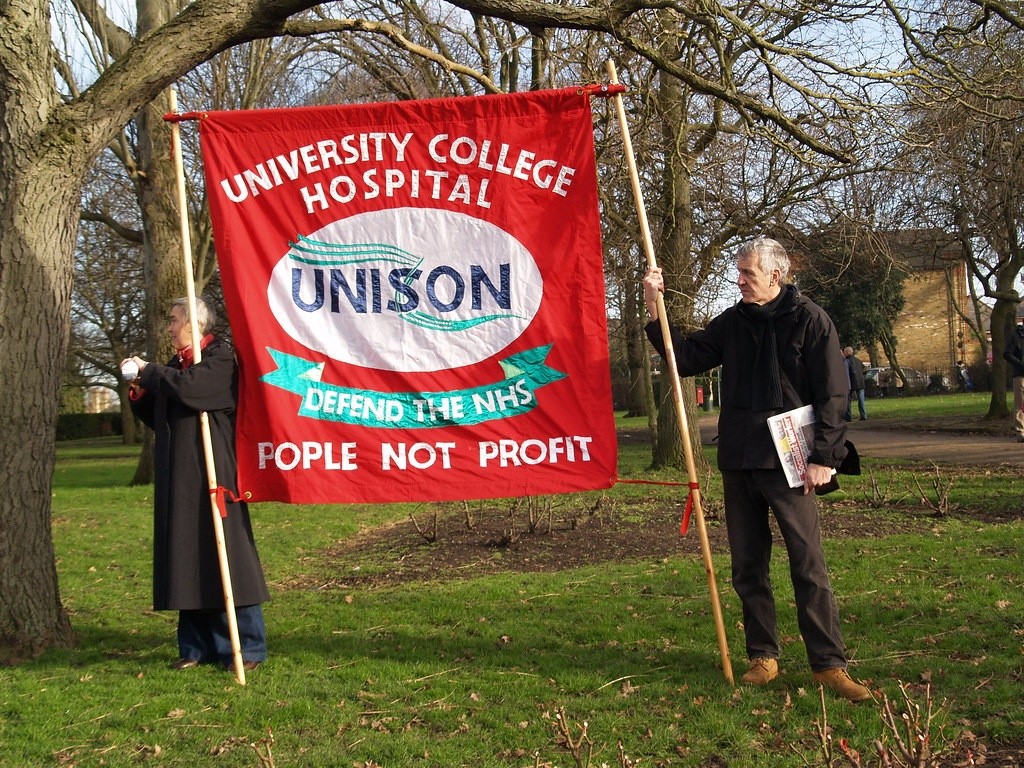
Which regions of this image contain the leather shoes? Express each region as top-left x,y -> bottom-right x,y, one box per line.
226,660 -> 260,673
168,657 -> 200,671
741,657 -> 779,687
813,667 -> 870,702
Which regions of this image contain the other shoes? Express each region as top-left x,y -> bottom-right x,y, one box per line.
859,418 -> 867,421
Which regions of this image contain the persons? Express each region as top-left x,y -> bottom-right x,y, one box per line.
642,238 -> 876,704
877,367 -> 906,399
840,347 -> 868,422
1002,318 -> 1024,443
119,294 -> 275,672
957,360 -> 964,383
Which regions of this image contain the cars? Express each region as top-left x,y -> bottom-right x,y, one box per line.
864,367 -> 950,400
